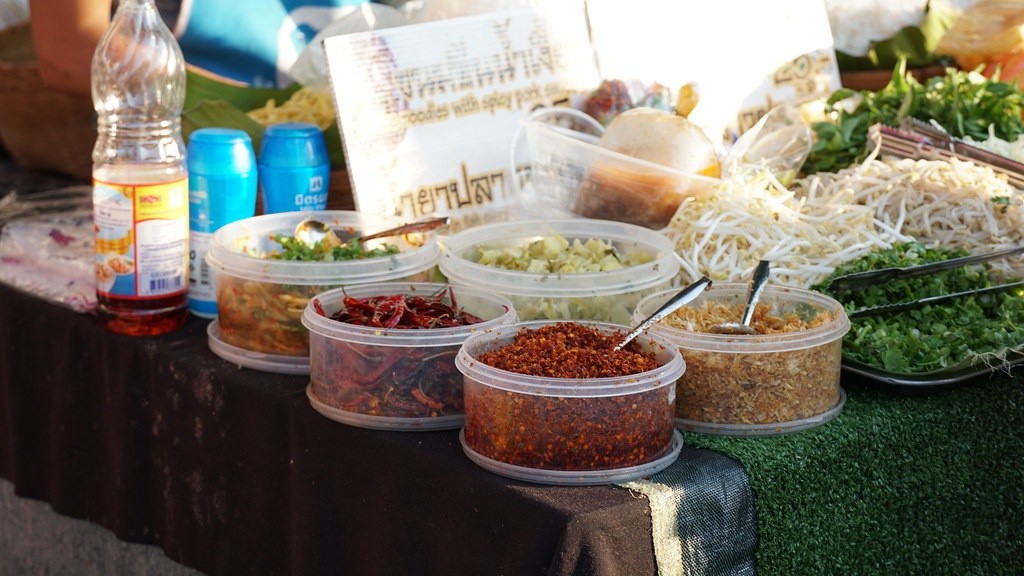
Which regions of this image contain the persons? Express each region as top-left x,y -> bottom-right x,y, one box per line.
30,0 -> 496,96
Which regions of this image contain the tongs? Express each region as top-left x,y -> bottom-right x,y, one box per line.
825,245 -> 1024,318
866,116 -> 1024,191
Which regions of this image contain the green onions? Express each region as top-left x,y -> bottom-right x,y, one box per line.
269,235 -> 400,261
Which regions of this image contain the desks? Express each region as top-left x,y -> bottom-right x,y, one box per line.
0,154 -> 1024,576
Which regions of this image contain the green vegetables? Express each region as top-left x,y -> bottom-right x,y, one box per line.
808,241 -> 1024,372
801,55 -> 1024,172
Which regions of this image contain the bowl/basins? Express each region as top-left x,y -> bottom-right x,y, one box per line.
438,218 -> 682,325
633,281 -> 851,437
300,281 -> 521,433
525,119 -> 724,230
454,319 -> 684,486
205,209 -> 442,375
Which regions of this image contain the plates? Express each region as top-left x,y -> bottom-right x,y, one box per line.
841,352 -> 1024,387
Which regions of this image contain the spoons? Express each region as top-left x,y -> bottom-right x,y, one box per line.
709,259 -> 771,336
294,217 -> 448,252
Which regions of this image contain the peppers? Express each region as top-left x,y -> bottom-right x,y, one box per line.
304,284 -> 486,416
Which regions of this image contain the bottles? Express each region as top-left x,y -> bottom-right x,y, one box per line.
259,122 -> 331,214
184,126 -> 259,319
89,0 -> 189,337
